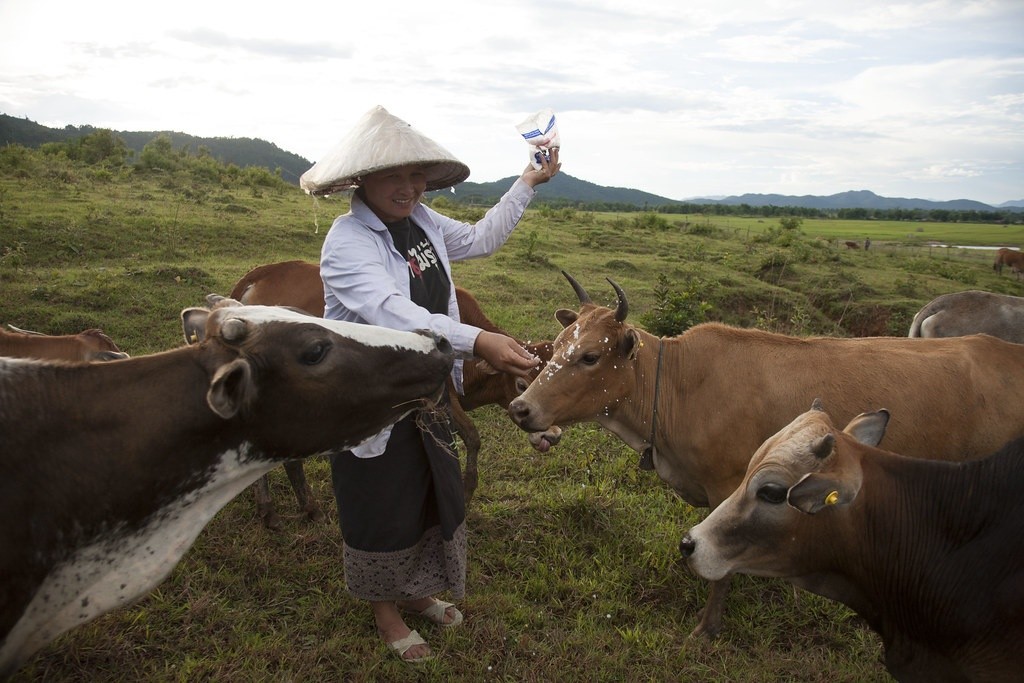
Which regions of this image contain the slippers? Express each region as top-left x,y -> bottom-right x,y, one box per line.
376,625 -> 436,663
397,598 -> 464,628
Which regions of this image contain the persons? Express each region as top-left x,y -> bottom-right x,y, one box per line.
300,106 -> 561,663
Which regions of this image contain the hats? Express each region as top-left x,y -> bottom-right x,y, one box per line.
300,105 -> 470,196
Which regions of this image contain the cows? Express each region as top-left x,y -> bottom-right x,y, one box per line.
0,242 -> 1023,683
